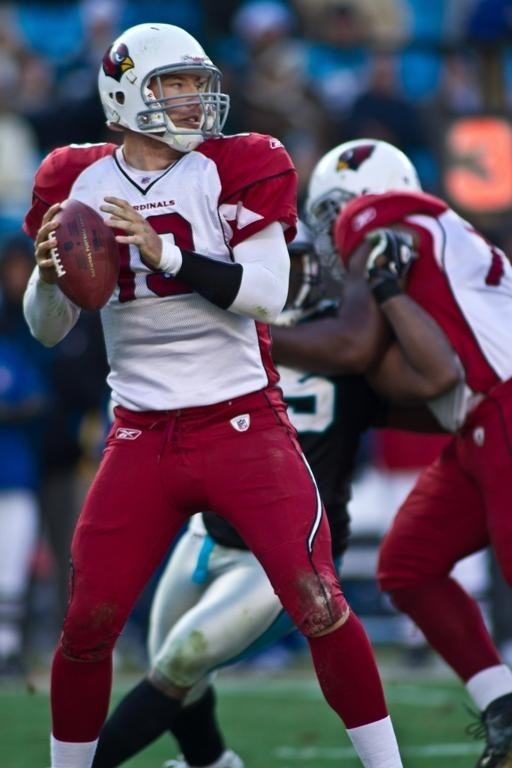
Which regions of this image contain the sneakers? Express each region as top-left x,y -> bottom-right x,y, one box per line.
462,691 -> 511,767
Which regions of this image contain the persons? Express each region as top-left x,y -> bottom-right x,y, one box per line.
92,211 -> 463,768
22,22 -> 402,768
269,139 -> 512,768
1,1 -> 512,677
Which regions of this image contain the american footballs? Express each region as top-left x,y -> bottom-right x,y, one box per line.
46,198 -> 120,312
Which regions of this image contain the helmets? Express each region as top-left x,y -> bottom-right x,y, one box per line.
281,218 -> 320,315
97,22 -> 230,153
302,137 -> 425,279
